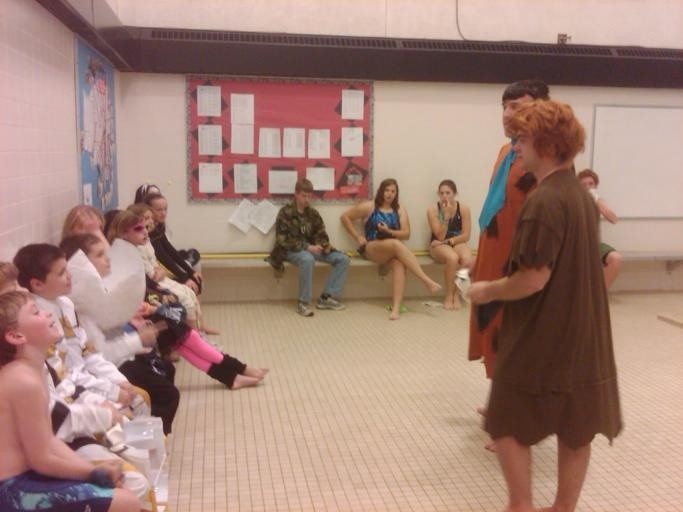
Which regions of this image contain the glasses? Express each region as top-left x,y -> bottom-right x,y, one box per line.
129,223 -> 150,231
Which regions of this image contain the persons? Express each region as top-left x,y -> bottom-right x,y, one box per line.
0,184 -> 270,512
340,178 -> 443,319
262,178 -> 352,317
466,79 -> 551,454
467,99 -> 622,511
576,169 -> 624,291
426,179 -> 473,309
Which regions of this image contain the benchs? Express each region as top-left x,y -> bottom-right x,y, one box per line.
189,247 -> 683,277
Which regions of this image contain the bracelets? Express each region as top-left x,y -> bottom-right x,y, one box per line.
448,237 -> 455,246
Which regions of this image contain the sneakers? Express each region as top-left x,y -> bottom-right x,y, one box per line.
296,301 -> 314,317
317,295 -> 346,311
